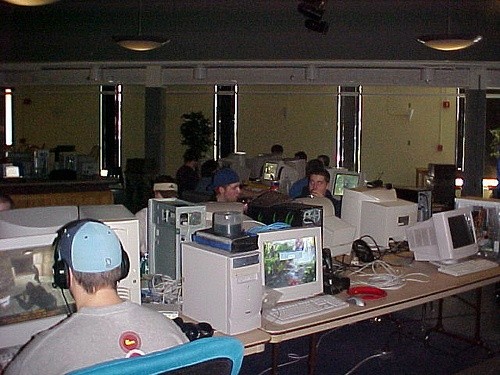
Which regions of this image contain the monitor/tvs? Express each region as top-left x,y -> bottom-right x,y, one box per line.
296,196 -> 357,257
406,207 -> 478,265
249,154 -> 277,180
331,172 -> 366,196
262,161 -> 286,181
218,153 -> 247,182
0,232 -> 77,326
255,226 -> 323,302
339,186 -> 418,251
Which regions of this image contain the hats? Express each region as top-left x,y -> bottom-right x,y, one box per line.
213,168 -> 240,186
56,221 -> 123,273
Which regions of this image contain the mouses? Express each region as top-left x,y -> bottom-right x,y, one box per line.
345,296 -> 366,307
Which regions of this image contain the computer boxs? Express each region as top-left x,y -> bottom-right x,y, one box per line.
289,158 -> 306,180
395,186 -> 432,220
454,196 -> 500,251
78,204 -> 142,305
148,197 -> 206,302
179,243 -> 262,335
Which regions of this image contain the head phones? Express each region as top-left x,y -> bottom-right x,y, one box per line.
322,273 -> 351,295
173,318 -> 213,341
351,235 -> 381,263
53,217 -> 129,289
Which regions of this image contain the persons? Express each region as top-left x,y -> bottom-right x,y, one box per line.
299,168 -> 341,218
197,159 -> 221,195
212,169 -> 249,212
318,155 -> 330,166
271,145 -> 283,156
294,151 -> 307,161
1,219 -> 191,375
176,149 -> 201,191
134,175 -> 183,257
290,160 -> 331,197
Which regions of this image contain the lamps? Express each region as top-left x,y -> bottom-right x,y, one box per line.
90,68 -> 103,81
420,68 -> 435,82
193,67 -> 207,80
297,0 -> 329,35
417,0 -> 483,51
306,67 -> 320,82
112,0 -> 171,51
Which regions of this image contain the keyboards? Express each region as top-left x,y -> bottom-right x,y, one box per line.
262,295 -> 349,325
438,258 -> 499,276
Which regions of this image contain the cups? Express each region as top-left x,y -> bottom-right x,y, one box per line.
483,190 -> 492,200
456,190 -> 461,198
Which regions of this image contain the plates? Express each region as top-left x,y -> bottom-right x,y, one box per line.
347,286 -> 387,300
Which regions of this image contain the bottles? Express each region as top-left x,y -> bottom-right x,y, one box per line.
140,253 -> 149,297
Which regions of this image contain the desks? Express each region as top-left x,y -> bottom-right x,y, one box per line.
259,251 -> 500,375
